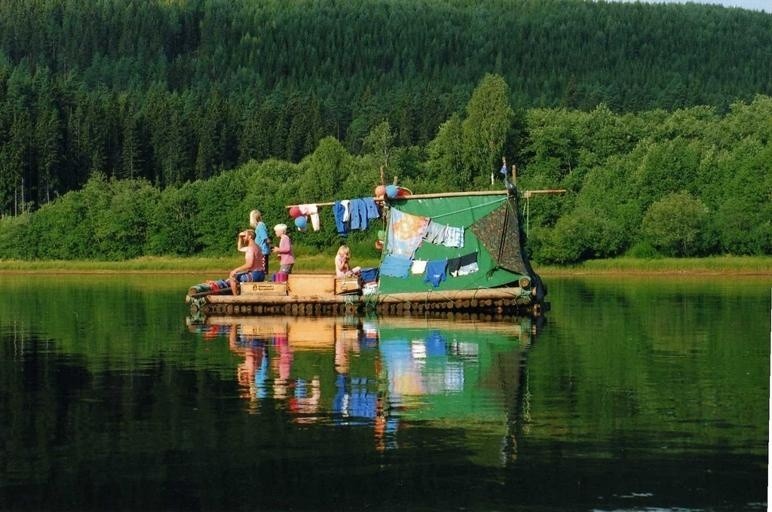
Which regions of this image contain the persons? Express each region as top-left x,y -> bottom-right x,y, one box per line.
229,209 -> 295,296
335,245 -> 361,279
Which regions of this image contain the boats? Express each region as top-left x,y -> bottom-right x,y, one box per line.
185,157 -> 567,317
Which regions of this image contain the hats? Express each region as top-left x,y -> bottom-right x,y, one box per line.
374,185 -> 397,198
289,207 -> 306,232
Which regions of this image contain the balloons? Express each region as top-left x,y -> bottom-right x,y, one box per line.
289,207 -> 306,228
375,185 -> 397,198
